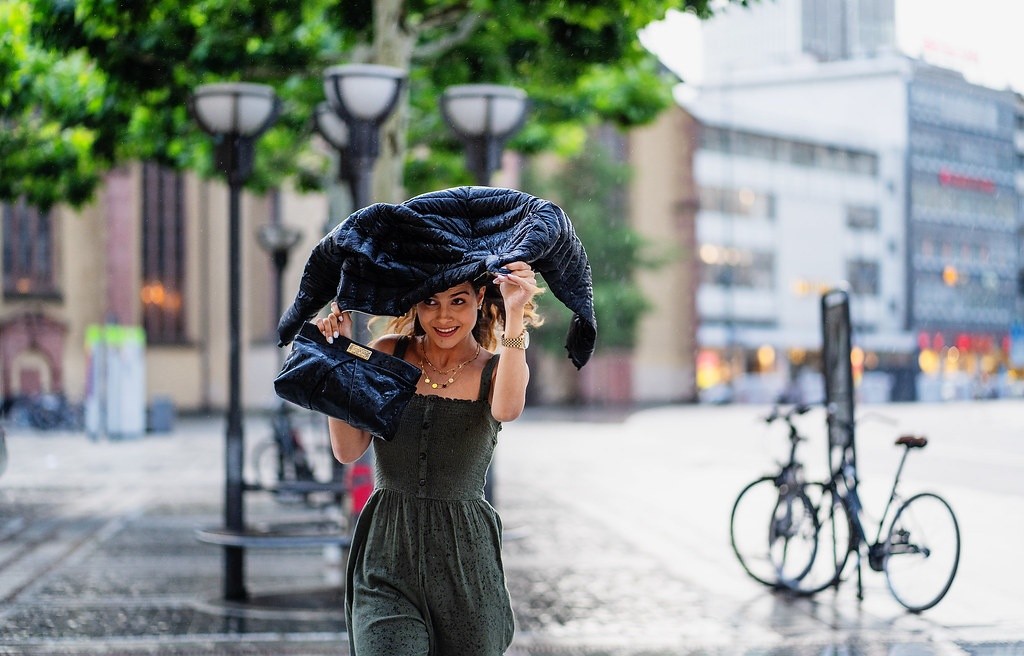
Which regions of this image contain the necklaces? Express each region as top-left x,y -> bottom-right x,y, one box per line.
418,334 -> 480,390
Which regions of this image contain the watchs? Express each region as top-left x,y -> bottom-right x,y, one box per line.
501,328 -> 530,351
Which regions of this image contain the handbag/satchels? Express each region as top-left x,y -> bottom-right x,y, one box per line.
274,322 -> 422,441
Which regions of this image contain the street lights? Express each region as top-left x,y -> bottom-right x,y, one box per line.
438,83 -> 529,190
188,79 -> 280,605
311,63 -> 411,508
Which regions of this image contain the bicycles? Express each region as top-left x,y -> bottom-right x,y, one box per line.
729,405 -> 963,615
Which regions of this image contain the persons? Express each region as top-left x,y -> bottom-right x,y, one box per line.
315,260 -> 539,656
